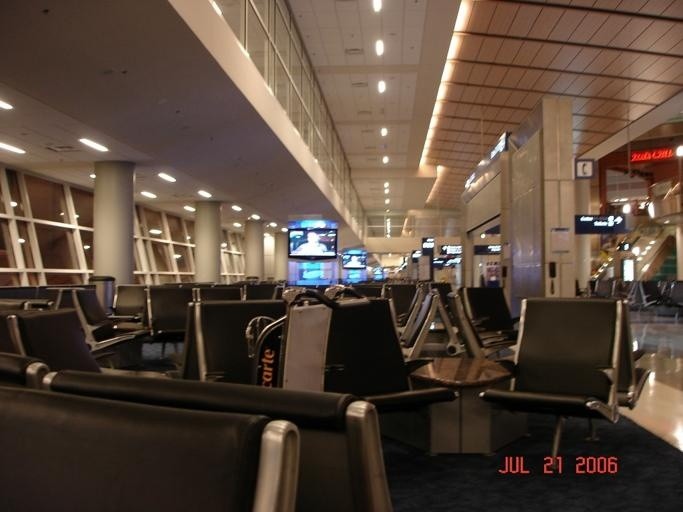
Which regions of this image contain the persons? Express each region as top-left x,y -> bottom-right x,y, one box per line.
343,255 -> 364,268
293,230 -> 328,255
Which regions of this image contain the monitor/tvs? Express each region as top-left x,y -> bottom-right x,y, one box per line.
287,229 -> 338,260
342,253 -> 367,269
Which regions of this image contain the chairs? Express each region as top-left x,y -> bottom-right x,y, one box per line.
1,277 -> 683,512
